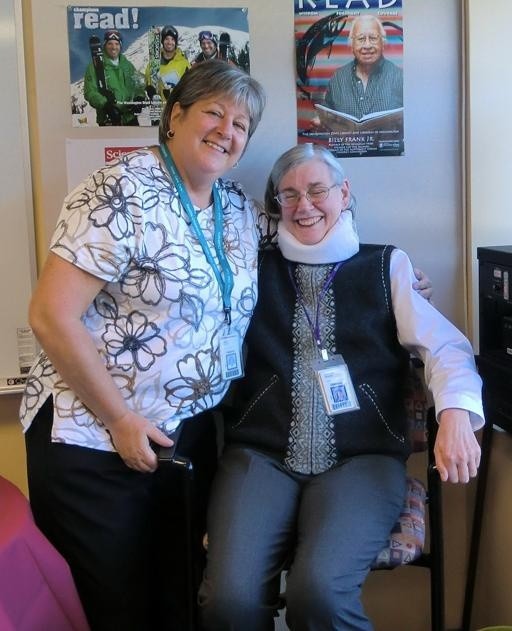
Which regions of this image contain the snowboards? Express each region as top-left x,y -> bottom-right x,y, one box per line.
219,33 -> 230,63
149,25 -> 163,125
90,35 -> 114,125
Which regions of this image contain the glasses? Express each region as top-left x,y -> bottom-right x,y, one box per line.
274,183 -> 337,208
105,31 -> 120,40
198,31 -> 213,40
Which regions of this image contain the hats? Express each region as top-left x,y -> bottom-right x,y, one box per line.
161,25 -> 178,44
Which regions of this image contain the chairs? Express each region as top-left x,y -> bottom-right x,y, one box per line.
172,357 -> 446,630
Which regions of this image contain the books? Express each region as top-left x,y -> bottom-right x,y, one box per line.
314,103 -> 403,140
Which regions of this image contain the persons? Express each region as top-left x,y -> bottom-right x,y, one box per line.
309,16 -> 403,133
84,30 -> 145,126
190,31 -> 235,65
145,24 -> 192,111
194,141 -> 487,631
20,60 -> 434,631
